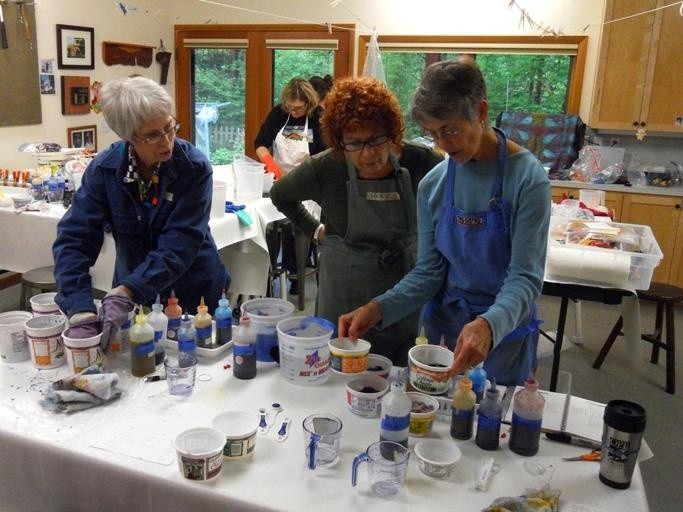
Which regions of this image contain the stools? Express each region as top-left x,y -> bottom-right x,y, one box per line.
20,265 -> 56,309
592,281 -> 683,394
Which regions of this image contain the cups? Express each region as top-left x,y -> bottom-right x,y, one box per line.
303,412 -> 343,473
351,440 -> 411,497
163,351 -> 197,396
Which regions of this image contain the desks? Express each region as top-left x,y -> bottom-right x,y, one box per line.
0,156 -> 309,294
539,202 -> 639,393
0,298 -> 647,512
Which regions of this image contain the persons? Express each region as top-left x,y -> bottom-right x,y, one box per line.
309,74 -> 333,109
254,76 -> 329,296
270,78 -> 446,367
338,60 -> 550,386
52,76 -> 230,355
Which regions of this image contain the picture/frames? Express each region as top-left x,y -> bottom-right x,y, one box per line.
67,125 -> 98,153
56,23 -> 95,70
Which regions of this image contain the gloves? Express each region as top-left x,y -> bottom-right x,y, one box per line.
260,154 -> 281,179
68,316 -> 99,339
99,295 -> 134,353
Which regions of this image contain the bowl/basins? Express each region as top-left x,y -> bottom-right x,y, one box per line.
405,392 -> 439,436
328,337 -> 370,376
369,353 -> 394,376
414,439 -> 461,481
12,196 -> 32,211
174,427 -> 227,483
212,411 -> 257,460
344,375 -> 389,420
407,344 -> 456,395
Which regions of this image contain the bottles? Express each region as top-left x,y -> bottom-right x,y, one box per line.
48,175 -> 57,202
57,172 -> 65,202
42,174 -> 48,200
475,377 -> 502,451
32,176 -> 42,199
509,368 -> 545,456
193,296 -> 213,350
450,378 -> 476,440
64,179 -> 71,209
215,288 -> 232,344
147,293 -> 168,365
177,309 -> 196,368
469,361 -> 487,415
232,308 -> 257,379
129,304 -> 155,377
379,370 -> 412,462
165,289 -> 182,341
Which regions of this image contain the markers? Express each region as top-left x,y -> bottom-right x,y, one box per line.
546,431 -> 602,449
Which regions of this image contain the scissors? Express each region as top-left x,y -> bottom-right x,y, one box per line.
561,448 -> 604,461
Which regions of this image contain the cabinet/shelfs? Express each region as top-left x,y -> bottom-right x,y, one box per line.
548,186 -> 683,287
578,0 -> 683,134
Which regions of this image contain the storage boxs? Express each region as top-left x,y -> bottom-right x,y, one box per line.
546,217 -> 664,290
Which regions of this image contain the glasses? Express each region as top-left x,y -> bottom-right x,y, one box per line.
339,133 -> 389,152
420,128 -> 458,142
285,102 -> 307,112
133,118 -> 181,145
